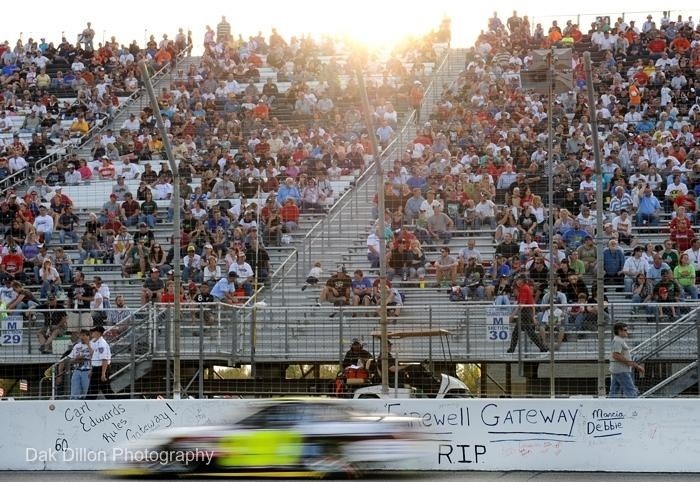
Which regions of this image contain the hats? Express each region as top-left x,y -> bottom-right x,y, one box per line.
90,325 -> 105,333
0,10 -> 700,302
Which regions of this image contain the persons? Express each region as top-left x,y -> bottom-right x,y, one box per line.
609,323 -> 646,397
341,338 -> 413,388
68,326 -> 115,400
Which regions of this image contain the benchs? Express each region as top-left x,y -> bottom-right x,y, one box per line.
694,220 -> 700,359
